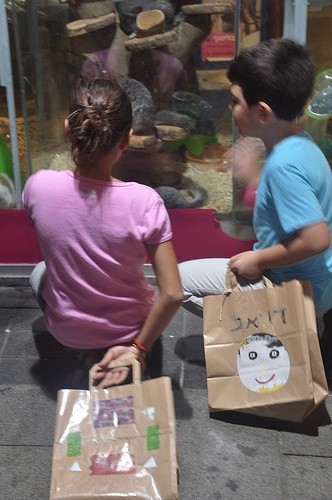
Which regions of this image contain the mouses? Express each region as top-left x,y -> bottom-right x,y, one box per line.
118,78 -> 156,134
154,184 -> 208,209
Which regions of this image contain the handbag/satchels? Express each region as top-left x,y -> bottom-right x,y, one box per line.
48,360 -> 191,497
202,268 -> 329,422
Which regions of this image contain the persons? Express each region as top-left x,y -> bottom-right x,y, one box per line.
177,40 -> 332,341
21,76 -> 182,389
76,13 -> 188,115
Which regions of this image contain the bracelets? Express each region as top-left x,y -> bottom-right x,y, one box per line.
130,338 -> 151,353
